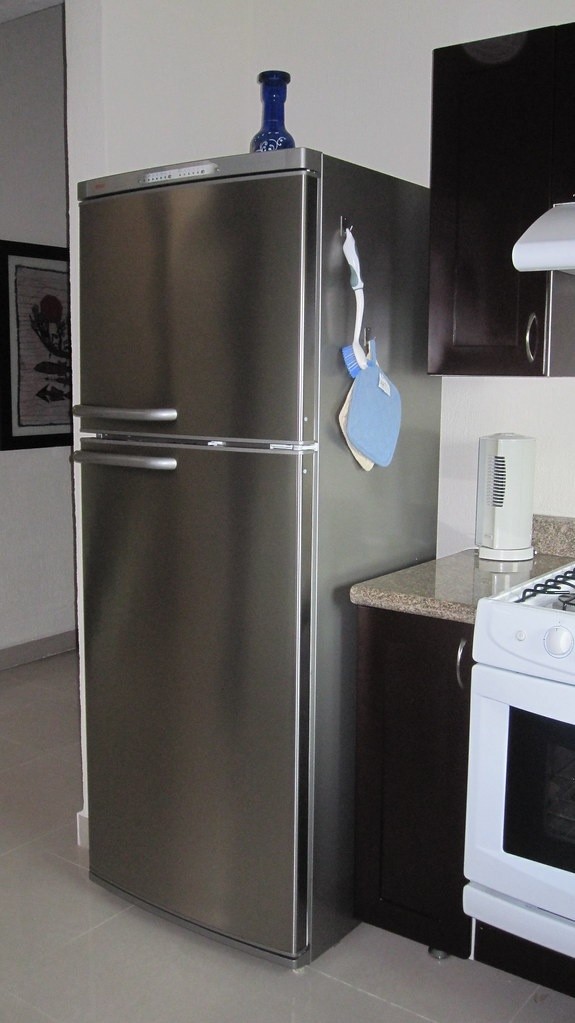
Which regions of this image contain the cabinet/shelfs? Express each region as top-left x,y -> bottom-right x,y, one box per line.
426,24 -> 574,378
355,604 -> 473,962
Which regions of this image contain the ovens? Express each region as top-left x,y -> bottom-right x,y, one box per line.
463,660 -> 575,964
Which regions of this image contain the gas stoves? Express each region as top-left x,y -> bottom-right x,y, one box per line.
470,554 -> 575,687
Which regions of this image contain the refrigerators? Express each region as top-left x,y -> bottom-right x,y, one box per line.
74,145 -> 438,975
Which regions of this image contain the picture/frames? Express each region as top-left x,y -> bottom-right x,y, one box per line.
0,240 -> 73,451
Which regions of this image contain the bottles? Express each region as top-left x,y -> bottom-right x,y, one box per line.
248,68 -> 295,153
